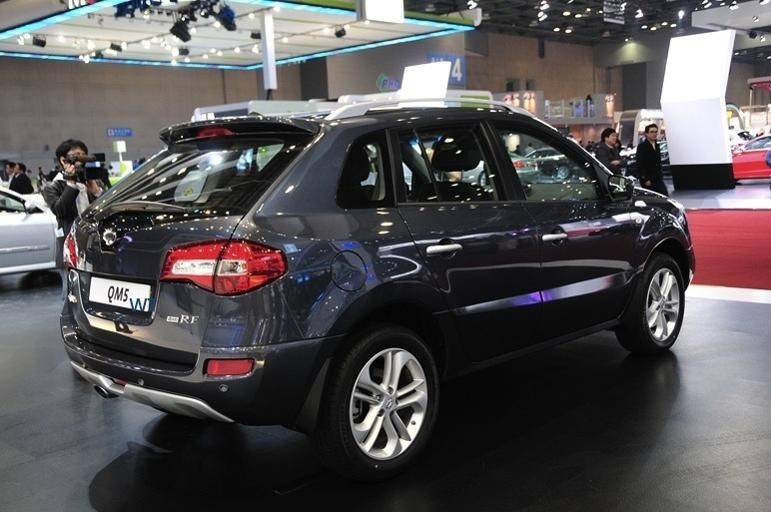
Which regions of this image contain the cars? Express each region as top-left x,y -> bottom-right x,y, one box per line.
527,144 -> 595,182
726,127 -> 771,181
619,136 -> 672,171
449,148 -> 541,188
0,185 -> 72,272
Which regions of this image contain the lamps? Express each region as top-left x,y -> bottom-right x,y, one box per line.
170,0 -> 242,42
24,37 -> 190,59
114,0 -> 173,22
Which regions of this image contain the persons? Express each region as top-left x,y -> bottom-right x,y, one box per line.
0,139 -> 113,301
512,94 -> 741,197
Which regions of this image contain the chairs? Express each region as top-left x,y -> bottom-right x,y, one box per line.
416,129 -> 491,200
335,144 -> 377,209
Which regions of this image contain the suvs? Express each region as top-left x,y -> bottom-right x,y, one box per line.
58,90 -> 701,479
